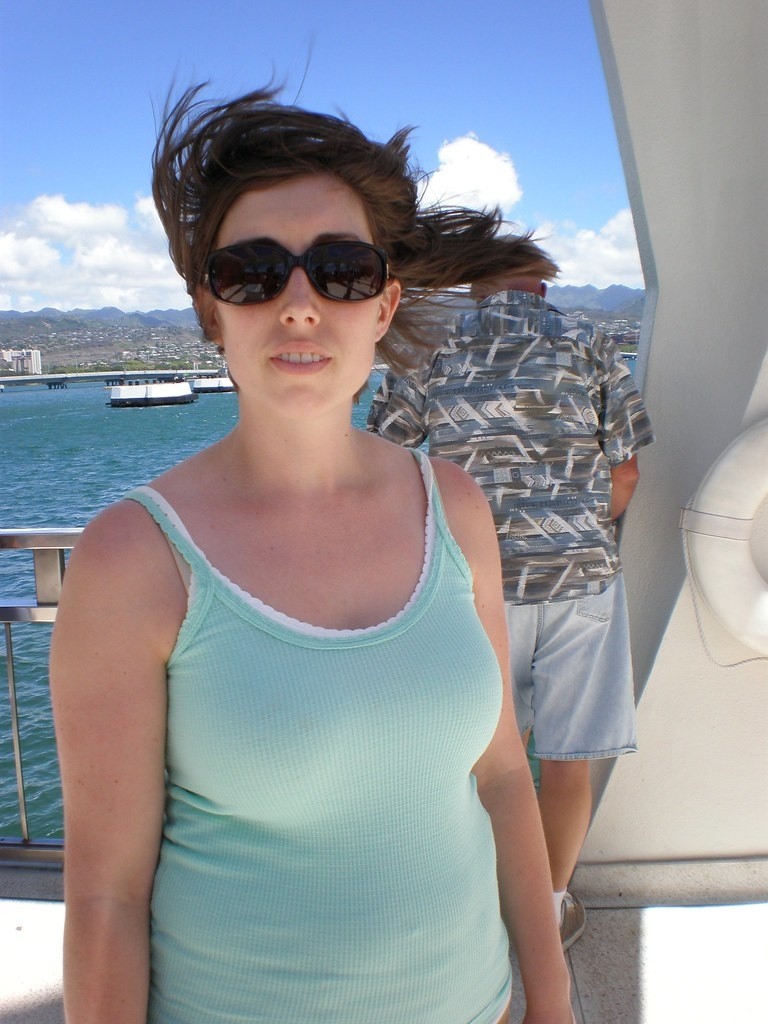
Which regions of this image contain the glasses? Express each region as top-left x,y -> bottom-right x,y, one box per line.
205,240 -> 389,306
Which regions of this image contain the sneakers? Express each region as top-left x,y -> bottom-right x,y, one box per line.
557,887 -> 587,959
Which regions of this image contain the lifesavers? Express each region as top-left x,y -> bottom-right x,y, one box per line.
676,417 -> 768,661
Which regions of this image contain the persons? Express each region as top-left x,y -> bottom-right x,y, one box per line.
362,234 -> 655,955
47,72 -> 571,1023
